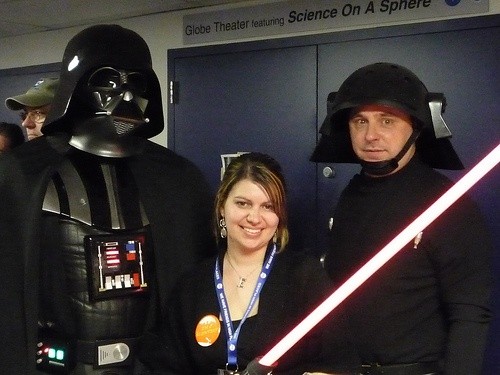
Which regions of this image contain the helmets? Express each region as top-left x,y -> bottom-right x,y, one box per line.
40,23 -> 166,160
308,61 -> 465,170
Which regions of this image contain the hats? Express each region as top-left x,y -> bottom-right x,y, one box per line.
5,76 -> 61,111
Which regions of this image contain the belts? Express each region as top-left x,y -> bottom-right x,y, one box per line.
75,334 -> 154,367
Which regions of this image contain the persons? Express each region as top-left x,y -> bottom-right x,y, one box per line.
155,154 -> 355,375
309,62 -> 491,375
0,25 -> 212,375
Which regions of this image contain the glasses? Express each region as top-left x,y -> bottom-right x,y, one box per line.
22,112 -> 47,123
